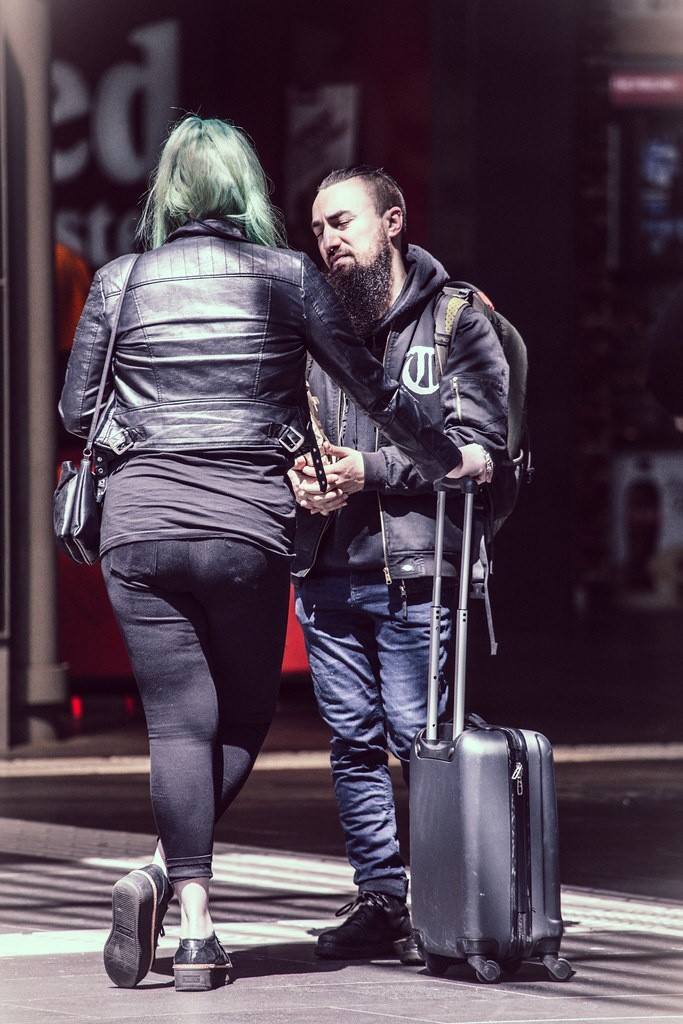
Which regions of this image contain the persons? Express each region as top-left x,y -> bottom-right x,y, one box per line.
285,164 -> 514,964
60,118 -> 495,991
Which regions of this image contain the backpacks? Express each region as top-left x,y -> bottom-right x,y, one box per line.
432,286 -> 526,541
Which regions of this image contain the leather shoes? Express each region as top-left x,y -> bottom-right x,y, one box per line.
172,933 -> 236,990
314,889 -> 412,959
104,864 -> 173,988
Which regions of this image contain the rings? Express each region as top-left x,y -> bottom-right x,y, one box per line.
487,460 -> 494,474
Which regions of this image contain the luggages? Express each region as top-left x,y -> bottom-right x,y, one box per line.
411,476 -> 577,983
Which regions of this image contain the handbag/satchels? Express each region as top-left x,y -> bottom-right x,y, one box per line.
51,459 -> 101,568
